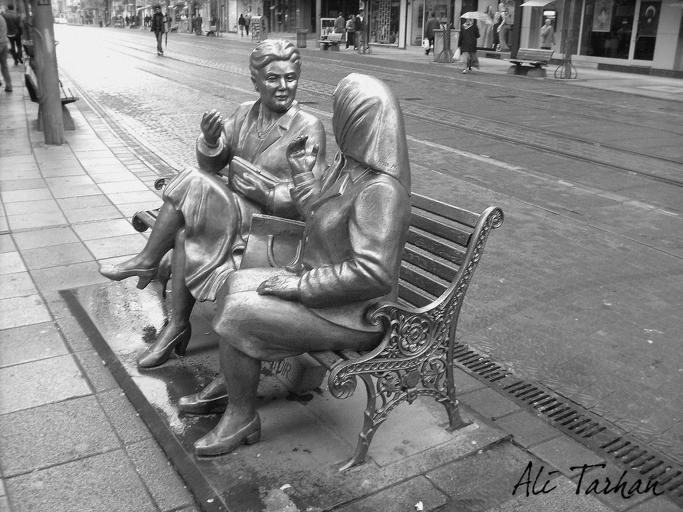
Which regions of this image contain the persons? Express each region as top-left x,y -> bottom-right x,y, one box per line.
238,14 -> 245,25
539,18 -> 556,50
424,11 -> 440,56
333,8 -> 364,49
482,2 -> 511,52
99,38 -> 328,369
150,4 -> 165,55
167,11 -> 202,33
0,15 -> 13,92
125,13 -> 152,27
458,18 -> 480,74
175,75 -> 411,458
244,15 -> 250,35
4,3 -> 23,65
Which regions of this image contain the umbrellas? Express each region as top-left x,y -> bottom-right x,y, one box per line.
460,11 -> 491,22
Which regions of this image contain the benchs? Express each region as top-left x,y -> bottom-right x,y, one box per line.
131,173 -> 506,471
502,47 -> 554,77
317,33 -> 343,51
24,56 -> 79,130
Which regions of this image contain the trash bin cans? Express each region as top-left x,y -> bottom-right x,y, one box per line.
296,26 -> 309,48
